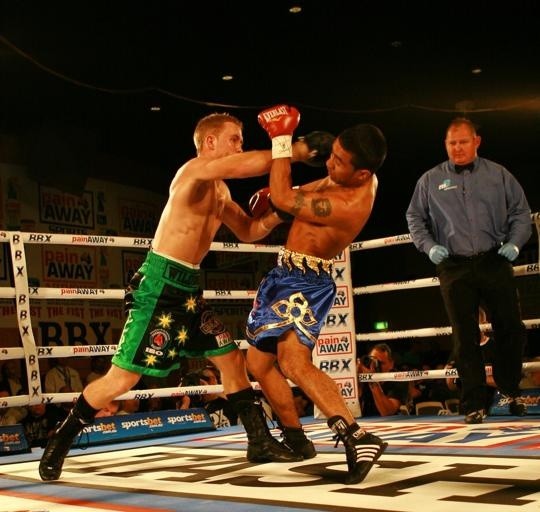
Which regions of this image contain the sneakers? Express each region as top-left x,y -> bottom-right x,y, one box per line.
509,397 -> 527,417
464,408 -> 488,424
276,420 -> 317,459
327,415 -> 388,484
39,403 -> 95,481
234,401 -> 303,462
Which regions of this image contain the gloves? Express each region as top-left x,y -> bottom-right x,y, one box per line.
249,185 -> 300,222
258,105 -> 301,140
498,242 -> 520,261
428,245 -> 448,265
298,131 -> 336,168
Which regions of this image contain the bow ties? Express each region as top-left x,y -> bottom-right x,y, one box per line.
455,163 -> 476,175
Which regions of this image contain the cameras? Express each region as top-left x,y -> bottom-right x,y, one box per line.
360,354 -> 381,372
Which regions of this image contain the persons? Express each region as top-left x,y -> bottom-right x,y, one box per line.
406,120 -> 531,425
39,114 -> 342,481
9,340 -> 540,419
250,106 -> 388,485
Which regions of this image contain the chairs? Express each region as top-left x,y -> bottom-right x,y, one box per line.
416,398 -> 460,417
